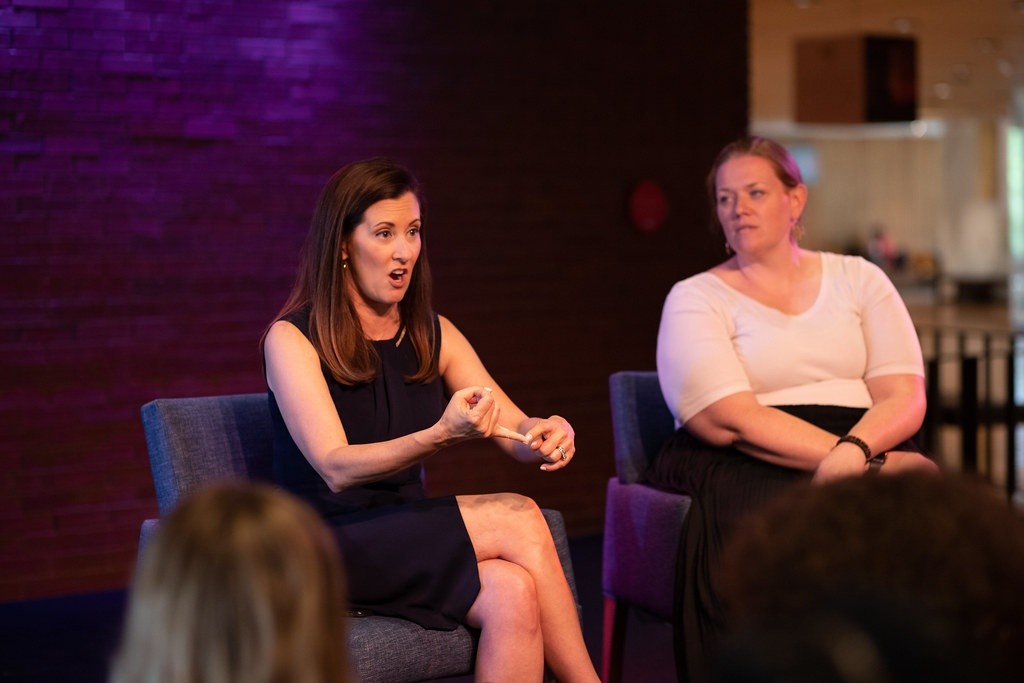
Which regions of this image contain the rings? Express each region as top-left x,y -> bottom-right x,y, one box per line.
556,446 -> 566,460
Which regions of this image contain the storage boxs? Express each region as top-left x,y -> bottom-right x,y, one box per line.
789,33 -> 919,127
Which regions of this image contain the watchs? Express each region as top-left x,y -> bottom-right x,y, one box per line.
868,451 -> 887,470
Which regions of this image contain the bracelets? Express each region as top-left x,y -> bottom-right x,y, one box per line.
836,435 -> 871,459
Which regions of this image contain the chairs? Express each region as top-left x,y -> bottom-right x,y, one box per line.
136,393 -> 576,683
602,371 -> 707,683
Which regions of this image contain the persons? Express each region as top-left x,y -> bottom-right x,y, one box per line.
647,134 -> 937,683
690,467 -> 1024,683
259,158 -> 600,683
111,482 -> 346,683
